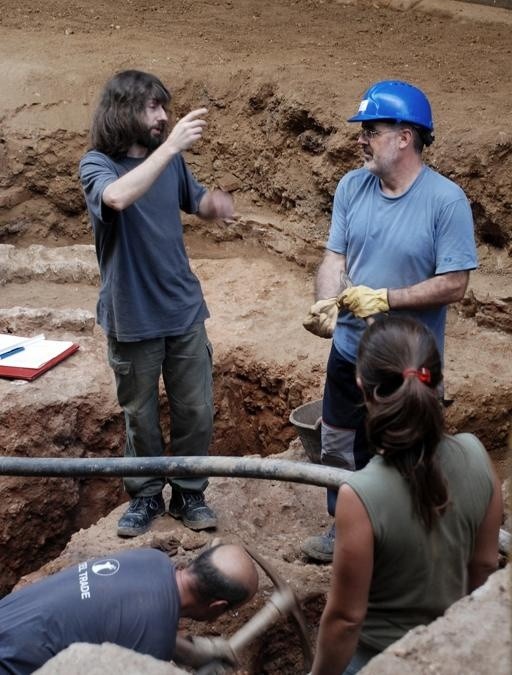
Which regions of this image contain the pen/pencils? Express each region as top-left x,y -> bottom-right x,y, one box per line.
0,345 -> 26,359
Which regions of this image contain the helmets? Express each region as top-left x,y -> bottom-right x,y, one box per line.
348,80 -> 433,132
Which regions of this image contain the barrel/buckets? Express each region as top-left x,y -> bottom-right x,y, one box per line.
290,399 -> 323,464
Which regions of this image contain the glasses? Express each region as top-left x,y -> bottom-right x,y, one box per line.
354,128 -> 392,138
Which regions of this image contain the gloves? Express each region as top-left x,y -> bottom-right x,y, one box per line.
340,284 -> 389,320
304,300 -> 339,337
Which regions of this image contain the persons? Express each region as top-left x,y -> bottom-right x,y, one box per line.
300,81 -> 478,560
0,543 -> 259,674
309,320 -> 503,674
78,71 -> 239,538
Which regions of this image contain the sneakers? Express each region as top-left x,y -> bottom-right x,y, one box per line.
117,492 -> 164,537
300,521 -> 337,560
168,492 -> 218,533
